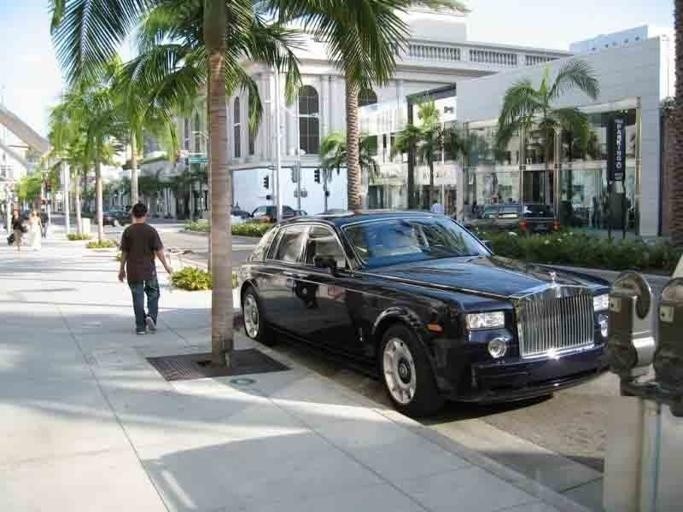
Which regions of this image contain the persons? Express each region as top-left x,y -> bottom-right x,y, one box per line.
430,198 -> 444,213
119,203 -> 172,335
26,211 -> 42,250
11,207 -> 23,253
41,208 -> 49,238
459,195 -> 517,219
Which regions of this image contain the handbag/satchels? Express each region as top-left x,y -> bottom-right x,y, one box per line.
21,224 -> 29,233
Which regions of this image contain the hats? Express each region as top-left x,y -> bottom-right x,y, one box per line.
12,207 -> 19,213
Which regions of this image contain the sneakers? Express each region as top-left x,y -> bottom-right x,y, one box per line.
137,315 -> 156,334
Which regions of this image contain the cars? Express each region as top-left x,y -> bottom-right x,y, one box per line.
93,210 -> 132,228
